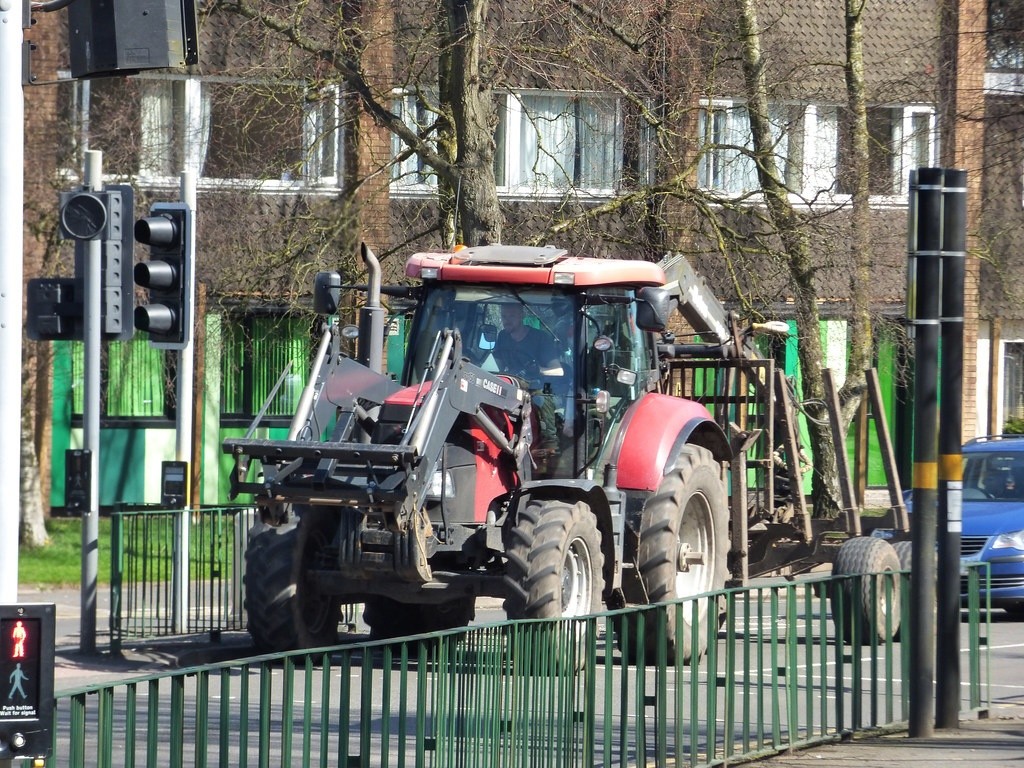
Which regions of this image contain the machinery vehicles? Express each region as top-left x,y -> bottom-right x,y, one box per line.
222,242 -> 913,676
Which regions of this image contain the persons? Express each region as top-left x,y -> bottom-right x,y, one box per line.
981,470 -> 1014,499
492,302 -> 564,457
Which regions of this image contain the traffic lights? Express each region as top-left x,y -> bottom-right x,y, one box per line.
0,603 -> 56,760
134,202 -> 192,350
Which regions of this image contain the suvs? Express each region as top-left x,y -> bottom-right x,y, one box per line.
870,434 -> 1024,622
